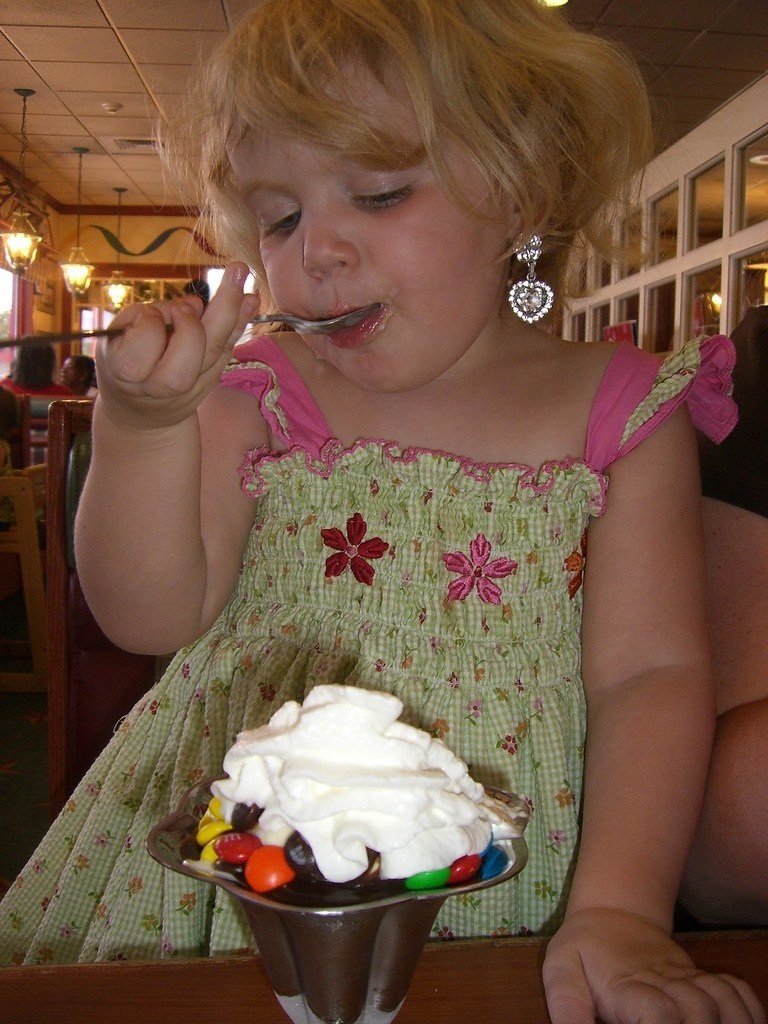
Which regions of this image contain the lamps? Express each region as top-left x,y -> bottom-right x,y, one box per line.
0,87 -> 46,276
103,187 -> 134,308
59,147 -> 99,298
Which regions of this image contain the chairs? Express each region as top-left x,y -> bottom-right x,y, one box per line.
0,384 -> 156,825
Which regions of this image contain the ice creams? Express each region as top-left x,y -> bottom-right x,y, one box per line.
193,682 -> 532,892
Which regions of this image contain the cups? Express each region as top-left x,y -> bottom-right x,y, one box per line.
146,784 -> 529,1024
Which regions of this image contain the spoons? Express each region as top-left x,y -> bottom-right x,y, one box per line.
0,302 -> 381,352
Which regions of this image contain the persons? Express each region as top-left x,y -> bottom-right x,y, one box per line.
181,279 -> 209,309
70,1 -> 768,1024
59,356 -> 98,399
0,336 -> 73,396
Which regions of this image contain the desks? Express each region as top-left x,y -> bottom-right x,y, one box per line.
0,928 -> 768,1024
